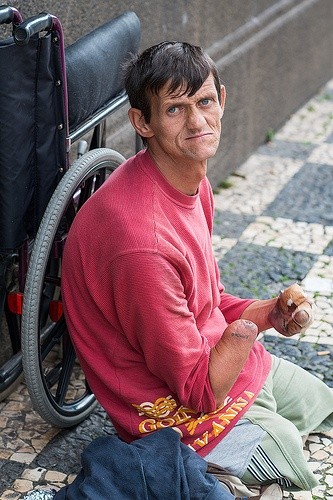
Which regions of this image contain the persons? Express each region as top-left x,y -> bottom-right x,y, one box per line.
57,38 -> 333,491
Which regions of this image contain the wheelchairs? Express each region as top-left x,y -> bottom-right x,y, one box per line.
0,6 -> 144,431
